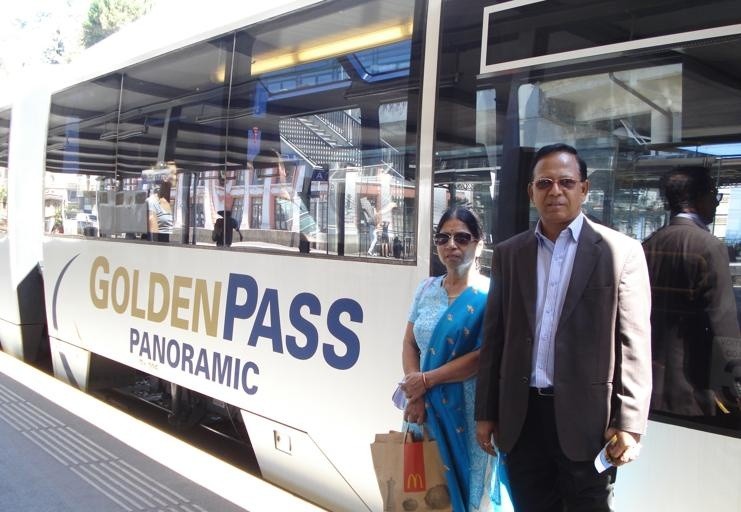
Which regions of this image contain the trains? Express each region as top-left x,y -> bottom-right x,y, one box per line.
0,0 -> 741,512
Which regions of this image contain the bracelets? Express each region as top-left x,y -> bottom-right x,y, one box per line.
422,372 -> 430,391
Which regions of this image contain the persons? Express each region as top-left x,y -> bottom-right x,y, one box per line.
215,210 -> 243,246
474,144 -> 652,511
141,158 -> 178,242
379,221 -> 390,258
401,209 -> 517,511
253,149 -> 321,242
215,168 -> 236,211
640,165 -> 741,426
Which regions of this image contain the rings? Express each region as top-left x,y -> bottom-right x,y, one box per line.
483,441 -> 491,446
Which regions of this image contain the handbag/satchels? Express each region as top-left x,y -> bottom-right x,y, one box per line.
212,230 -> 220,241
370,422 -> 452,512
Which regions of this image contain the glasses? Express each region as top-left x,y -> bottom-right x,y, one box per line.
712,193 -> 723,202
535,178 -> 576,189
434,233 -> 471,246
